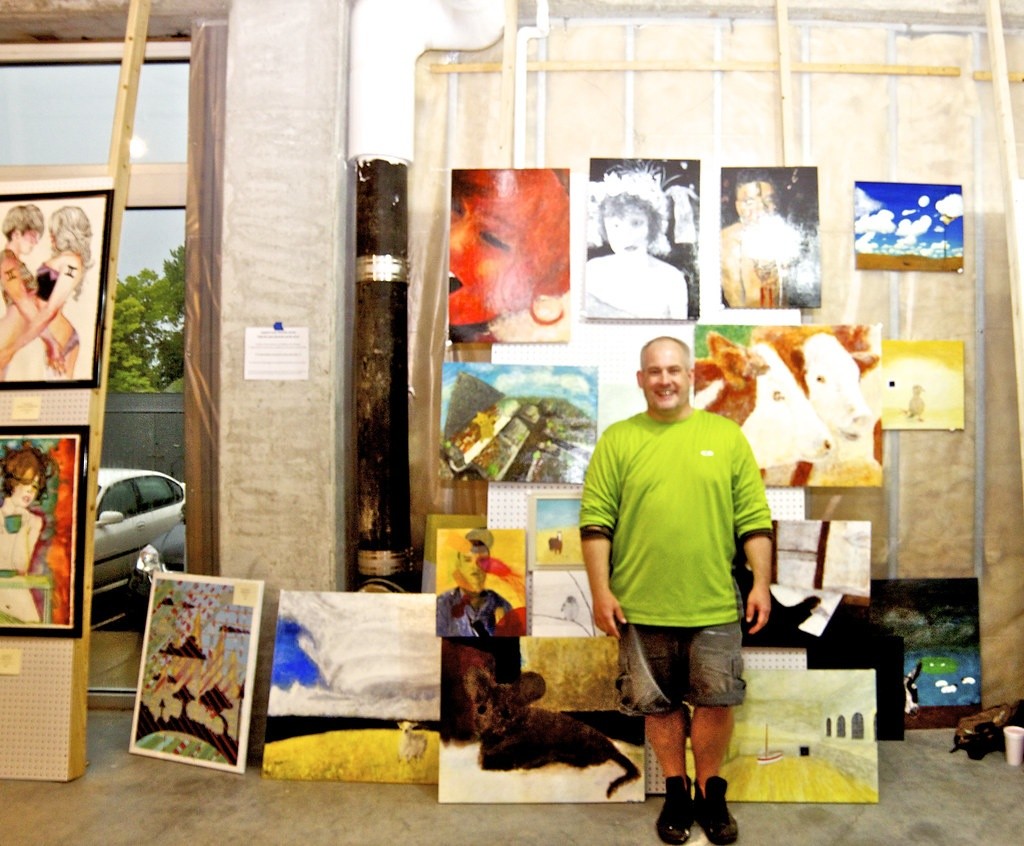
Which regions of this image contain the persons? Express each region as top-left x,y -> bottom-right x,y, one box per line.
579,337 -> 773,846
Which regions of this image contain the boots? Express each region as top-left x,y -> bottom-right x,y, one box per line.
656,775 -> 695,845
693,775 -> 738,846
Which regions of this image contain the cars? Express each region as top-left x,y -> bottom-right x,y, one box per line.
92,468 -> 186,598
127,514 -> 186,607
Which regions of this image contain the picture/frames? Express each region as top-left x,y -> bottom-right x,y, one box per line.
0,189 -> 115,390
1,423 -> 91,639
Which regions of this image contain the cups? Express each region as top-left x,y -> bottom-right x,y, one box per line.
1003,726 -> 1024,766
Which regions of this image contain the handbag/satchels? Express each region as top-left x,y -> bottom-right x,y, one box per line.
949,700 -> 1023,759
